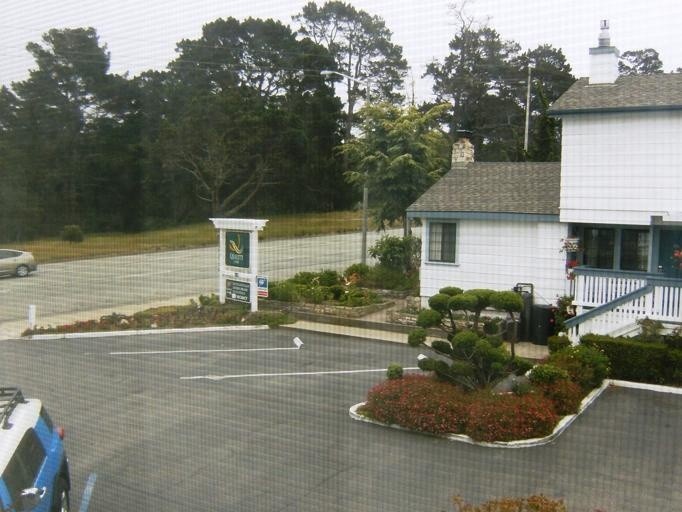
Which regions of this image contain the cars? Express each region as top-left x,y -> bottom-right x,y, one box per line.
0,248 -> 37,279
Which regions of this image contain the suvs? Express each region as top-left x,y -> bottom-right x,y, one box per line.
0,384 -> 72,512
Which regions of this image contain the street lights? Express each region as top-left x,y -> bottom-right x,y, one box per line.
321,69 -> 371,111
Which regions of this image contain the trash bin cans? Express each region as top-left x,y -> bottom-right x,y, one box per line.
532,304 -> 557,345
